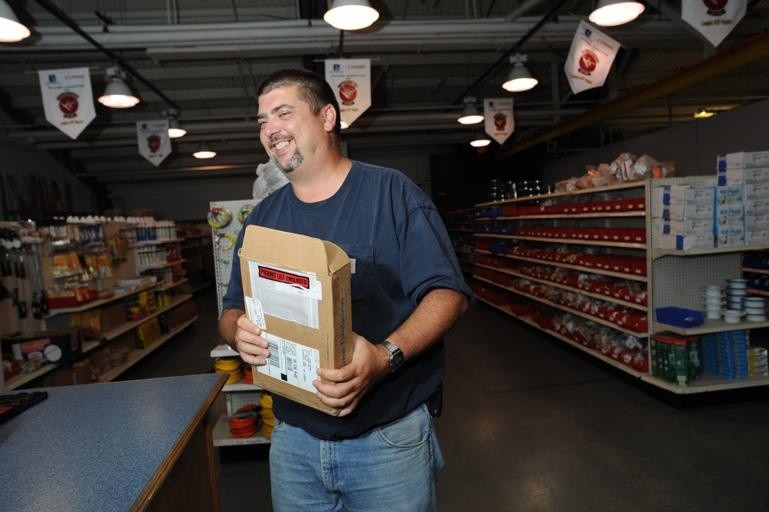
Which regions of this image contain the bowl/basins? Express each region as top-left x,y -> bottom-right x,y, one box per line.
701,279 -> 766,323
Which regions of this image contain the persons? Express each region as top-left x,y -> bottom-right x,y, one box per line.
218,69 -> 469,512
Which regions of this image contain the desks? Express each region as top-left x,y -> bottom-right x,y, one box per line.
0,374 -> 232,511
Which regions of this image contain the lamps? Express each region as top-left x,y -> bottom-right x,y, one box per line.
97,72 -> 142,108
501,52 -> 539,94
587,1 -> 645,30
322,0 -> 382,32
0,1 -> 33,45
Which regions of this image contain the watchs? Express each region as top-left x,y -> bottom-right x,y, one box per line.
381,340 -> 406,373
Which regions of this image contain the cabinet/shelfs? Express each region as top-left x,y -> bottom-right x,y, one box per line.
48,235 -> 212,381
210,344 -> 270,448
446,170 -> 769,396
